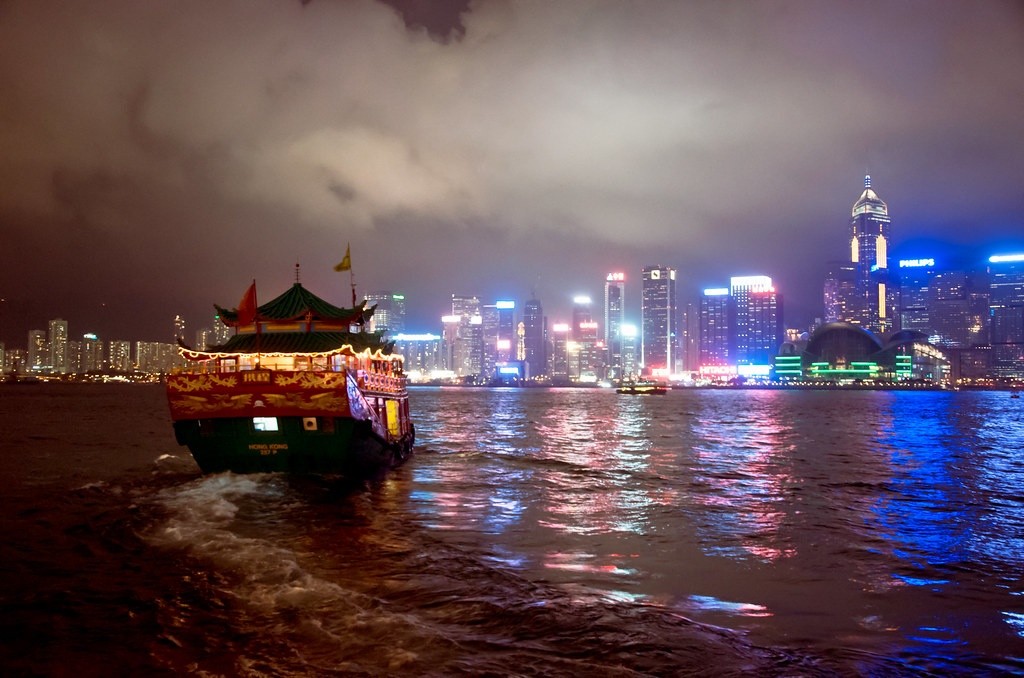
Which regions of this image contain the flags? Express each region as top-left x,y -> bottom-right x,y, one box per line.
238,280 -> 258,329
332,246 -> 351,271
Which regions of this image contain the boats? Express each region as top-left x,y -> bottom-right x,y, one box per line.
161,243 -> 415,477
613,373 -> 671,395
